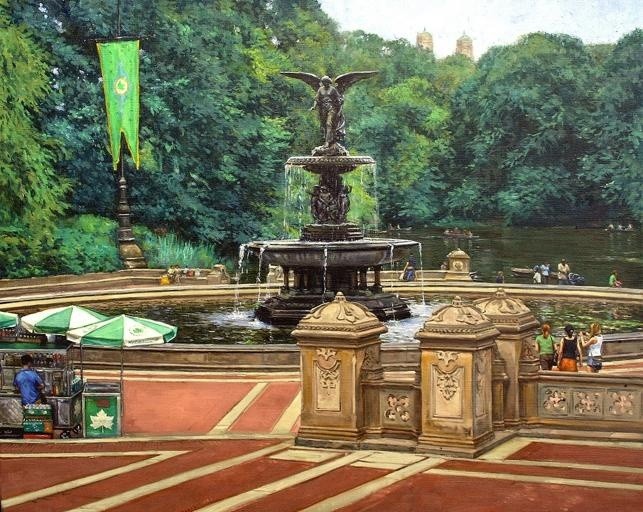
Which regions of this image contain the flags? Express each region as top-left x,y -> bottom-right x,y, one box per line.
94,38 -> 141,171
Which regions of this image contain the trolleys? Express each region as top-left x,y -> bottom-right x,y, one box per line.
0,375 -> 84,439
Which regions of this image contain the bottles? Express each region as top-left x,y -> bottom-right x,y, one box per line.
22,403 -> 54,434
0,328 -> 81,396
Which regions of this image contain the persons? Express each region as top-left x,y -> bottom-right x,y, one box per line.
533,324 -> 557,371
557,325 -> 583,372
608,270 -> 618,287
496,271 -> 504,283
13,354 -> 47,406
167,264 -> 201,286
311,76 -> 341,149
579,323 -> 603,373
403,253 -> 416,281
532,258 -> 570,284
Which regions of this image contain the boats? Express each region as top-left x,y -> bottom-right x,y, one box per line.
443,229 -> 479,240
387,227 -> 412,233
510,267 -> 558,279
601,227 -> 639,236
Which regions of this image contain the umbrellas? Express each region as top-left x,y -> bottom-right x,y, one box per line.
65,313 -> 178,437
0,310 -> 18,330
20,306 -> 109,397
